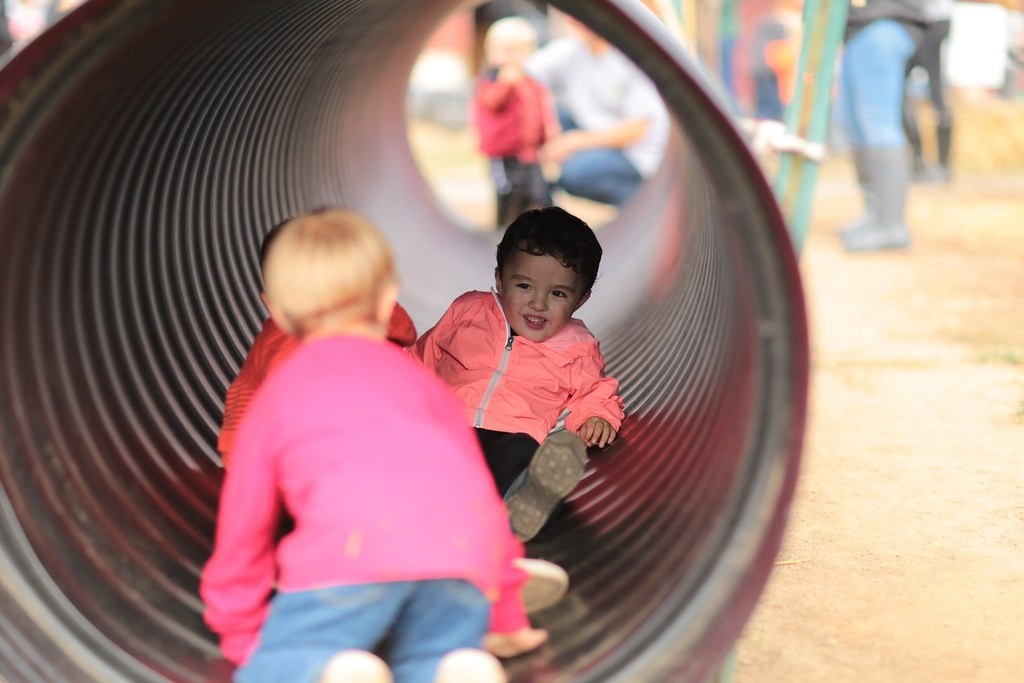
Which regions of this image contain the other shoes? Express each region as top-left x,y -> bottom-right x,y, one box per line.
506,432 -> 587,542
321,649 -> 393,683
514,556 -> 568,613
435,648 -> 507,683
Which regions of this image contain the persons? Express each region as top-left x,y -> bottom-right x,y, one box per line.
836,0 -> 929,256
902,0 -> 952,184
525,14 -> 671,206
423,207 -> 626,542
217,218 -> 434,472
198,210 -> 547,683
473,17 -> 562,228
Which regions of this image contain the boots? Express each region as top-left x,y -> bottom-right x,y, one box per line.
837,146 -> 912,252
